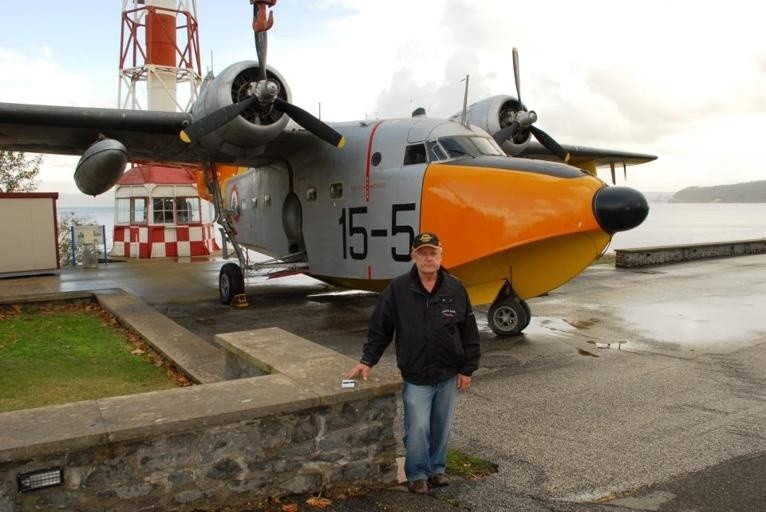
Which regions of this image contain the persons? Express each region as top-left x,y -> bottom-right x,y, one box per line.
345,229 -> 483,498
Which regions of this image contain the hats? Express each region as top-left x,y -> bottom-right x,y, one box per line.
412,233 -> 440,252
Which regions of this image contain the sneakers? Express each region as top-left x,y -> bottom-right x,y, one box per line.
434,474 -> 450,486
410,479 -> 428,494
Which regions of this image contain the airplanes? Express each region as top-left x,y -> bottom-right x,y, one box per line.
0,1 -> 659,339
